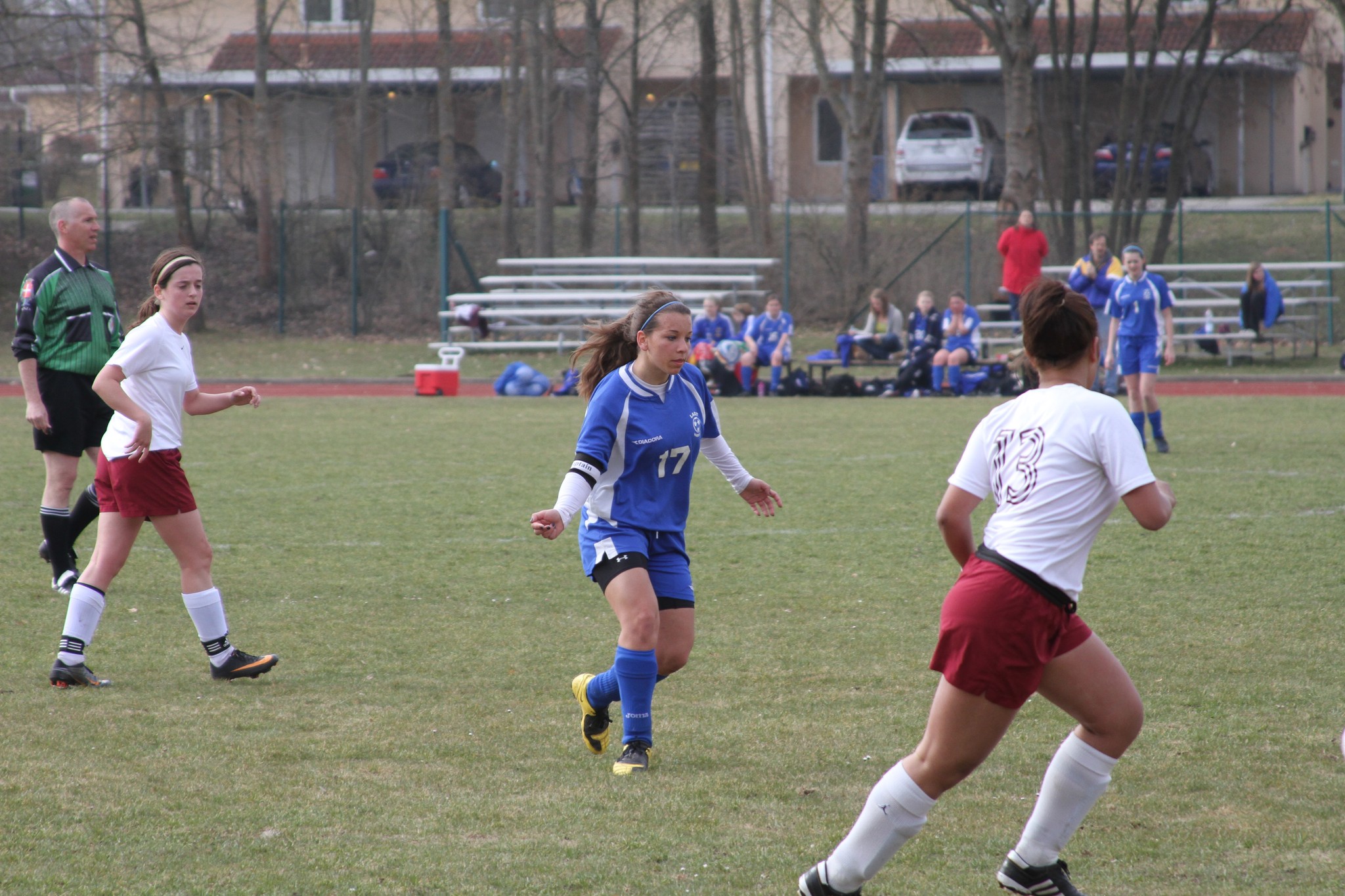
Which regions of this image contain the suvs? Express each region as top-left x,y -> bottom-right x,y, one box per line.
895,108 -> 1006,201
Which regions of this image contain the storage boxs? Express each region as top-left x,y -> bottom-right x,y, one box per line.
409,361 -> 458,398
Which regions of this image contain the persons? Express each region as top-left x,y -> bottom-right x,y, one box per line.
700,300 -> 757,396
881,289 -> 944,396
10,195 -> 125,594
690,297 -> 737,358
1237,261 -> 1284,334
530,290 -> 782,775
930,293 -> 982,394
848,287 -> 905,360
739,293 -> 791,394
996,209 -> 1051,335
795,278 -> 1175,895
1068,230 -> 1123,395
1104,244 -> 1172,453
50,248 -> 278,689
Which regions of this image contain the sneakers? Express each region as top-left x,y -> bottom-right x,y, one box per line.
51,569 -> 79,595
38,539 -> 78,567
612,742 -> 651,776
49,658 -> 111,689
997,850 -> 1089,896
1153,433 -> 1168,452
209,648 -> 278,683
571,673 -> 610,755
797,861 -> 863,896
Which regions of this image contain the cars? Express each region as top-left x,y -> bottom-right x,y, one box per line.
374,140 -> 503,207
1094,123 -> 1215,199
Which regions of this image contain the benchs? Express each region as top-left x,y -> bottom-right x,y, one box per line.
428,255 -> 784,359
803,254 -> 1345,392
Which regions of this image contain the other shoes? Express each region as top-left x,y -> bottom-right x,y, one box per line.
881,389 -> 900,398
1239,328 -> 1258,339
928,390 -> 942,396
1093,389 -> 1115,397
911,388 -> 923,397
768,388 -> 778,396
737,391 -> 753,397
949,388 -> 963,397
